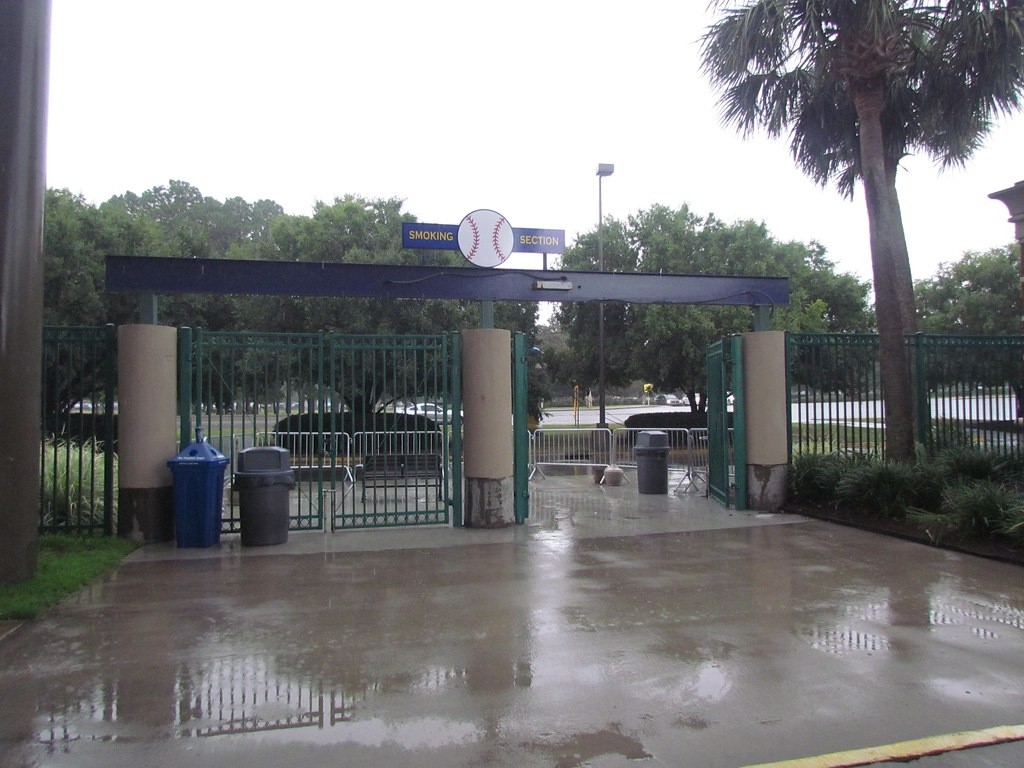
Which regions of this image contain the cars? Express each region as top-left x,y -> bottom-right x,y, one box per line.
654,392 -> 735,406
396,402 -> 464,424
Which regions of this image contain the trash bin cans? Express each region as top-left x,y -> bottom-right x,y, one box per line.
167,427 -> 232,548
633,431 -> 670,494
233,446 -> 294,546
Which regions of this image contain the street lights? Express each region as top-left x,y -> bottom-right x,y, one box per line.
596,162 -> 614,429
